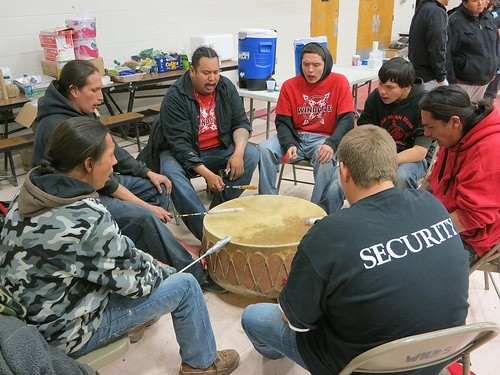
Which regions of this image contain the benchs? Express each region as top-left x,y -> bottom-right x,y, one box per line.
0,110 -> 145,188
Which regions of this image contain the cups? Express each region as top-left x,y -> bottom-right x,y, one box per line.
266,81 -> 276,92
362,60 -> 368,65
373,41 -> 378,50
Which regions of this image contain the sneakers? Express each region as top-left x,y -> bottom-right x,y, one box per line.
178,349 -> 241,375
200,273 -> 227,294
128,315 -> 161,342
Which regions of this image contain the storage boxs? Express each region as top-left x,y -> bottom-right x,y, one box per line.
40,26 -> 76,36
384,42 -> 409,58
89,56 -> 106,77
100,75 -> 111,86
43,47 -> 76,63
179,54 -> 190,70
110,73 -> 147,83
130,53 -> 181,74
361,60 -> 389,64
190,33 -> 234,63
39,34 -> 73,49
39,60 -> 70,81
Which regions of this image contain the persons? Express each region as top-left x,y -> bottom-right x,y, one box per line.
30,59 -> 231,296
405,0 -> 448,171
241,124 -> 470,375
257,43 -> 356,213
448,1 -> 500,112
0,116 -> 241,375
320,57 -> 440,216
127,46 -> 259,247
409,83 -> 500,283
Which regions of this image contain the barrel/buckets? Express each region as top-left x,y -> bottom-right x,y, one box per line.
237,27 -> 278,79
293,36 -> 328,76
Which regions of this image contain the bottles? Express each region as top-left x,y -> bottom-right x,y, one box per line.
352,55 -> 360,66
23,75 -> 32,97
4,76 -> 16,97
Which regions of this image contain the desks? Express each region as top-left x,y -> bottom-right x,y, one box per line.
234,64 -> 378,140
0,59 -> 239,182
343,50 -> 392,97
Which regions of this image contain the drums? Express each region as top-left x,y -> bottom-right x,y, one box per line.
200,195 -> 329,299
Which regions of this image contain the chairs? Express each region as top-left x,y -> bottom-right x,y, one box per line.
467,242 -> 500,301
336,321 -> 500,375
276,159 -> 315,195
0,312 -> 131,375
